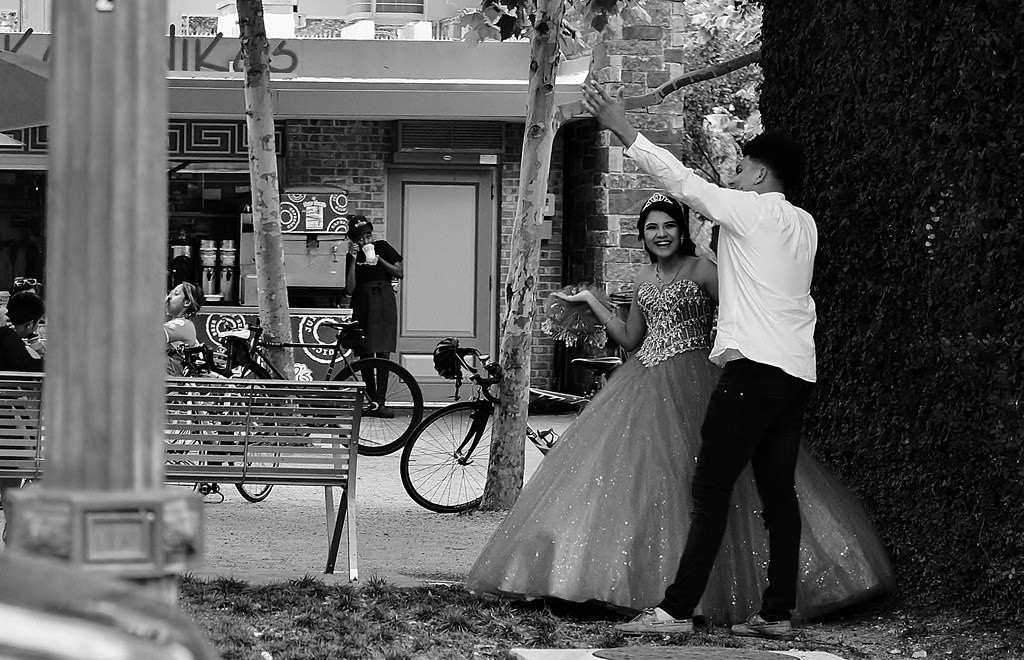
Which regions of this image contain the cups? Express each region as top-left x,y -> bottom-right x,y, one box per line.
0,290 -> 10,307
363,244 -> 375,264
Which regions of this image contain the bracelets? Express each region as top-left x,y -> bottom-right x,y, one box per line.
601,313 -> 617,331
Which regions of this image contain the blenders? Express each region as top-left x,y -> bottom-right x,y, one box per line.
200,239 -> 236,303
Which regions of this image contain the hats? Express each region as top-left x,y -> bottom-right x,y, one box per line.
347,215 -> 373,240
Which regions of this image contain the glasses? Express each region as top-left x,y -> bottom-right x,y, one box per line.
13,277 -> 37,291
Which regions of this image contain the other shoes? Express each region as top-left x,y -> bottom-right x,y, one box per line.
614,608 -> 693,636
362,402 -> 394,418
730,610 -> 794,640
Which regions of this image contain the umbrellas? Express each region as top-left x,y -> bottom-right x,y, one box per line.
0,49 -> 50,133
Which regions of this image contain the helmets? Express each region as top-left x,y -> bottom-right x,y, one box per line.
433,337 -> 462,379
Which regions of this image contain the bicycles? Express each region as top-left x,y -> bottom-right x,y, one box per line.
400,336 -> 624,514
166,318 -> 424,505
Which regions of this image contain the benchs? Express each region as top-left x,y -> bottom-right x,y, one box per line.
0,372 -> 365,582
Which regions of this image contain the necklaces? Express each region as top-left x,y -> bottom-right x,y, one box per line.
654,258 -> 687,292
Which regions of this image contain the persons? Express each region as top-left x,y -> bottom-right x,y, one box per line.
162,281 -> 205,395
345,215 -> 404,419
0,275 -> 46,470
463,192 -> 899,623
577,79 -> 819,641
